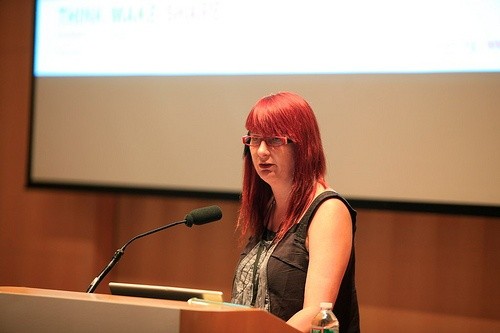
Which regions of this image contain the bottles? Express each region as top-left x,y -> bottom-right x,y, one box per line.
310,302 -> 339,333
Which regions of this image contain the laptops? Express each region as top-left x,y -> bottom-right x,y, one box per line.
109,282 -> 223,303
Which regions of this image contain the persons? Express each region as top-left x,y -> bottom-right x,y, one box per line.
231,93 -> 359,333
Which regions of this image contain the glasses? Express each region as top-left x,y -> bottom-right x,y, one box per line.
242,135 -> 295,148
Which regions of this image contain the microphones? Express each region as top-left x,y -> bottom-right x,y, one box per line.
86,206 -> 223,293
